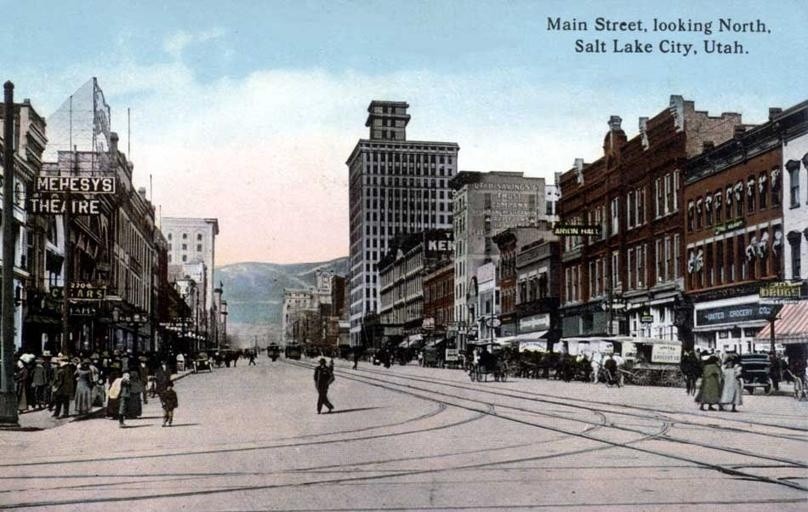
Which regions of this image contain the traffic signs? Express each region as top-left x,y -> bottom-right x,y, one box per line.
27,196 -> 101,218
33,175 -> 117,196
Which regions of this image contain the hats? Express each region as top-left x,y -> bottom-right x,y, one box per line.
37,347 -> 134,377
318,358 -> 326,365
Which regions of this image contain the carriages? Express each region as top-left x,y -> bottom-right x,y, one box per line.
679,350 -> 774,398
461,333 -> 685,389
203,346 -> 243,369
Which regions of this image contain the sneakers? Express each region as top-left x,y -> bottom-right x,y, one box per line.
161,422 -> 173,428
700,405 -> 740,412
19,400 -> 151,430
316,407 -> 334,415
